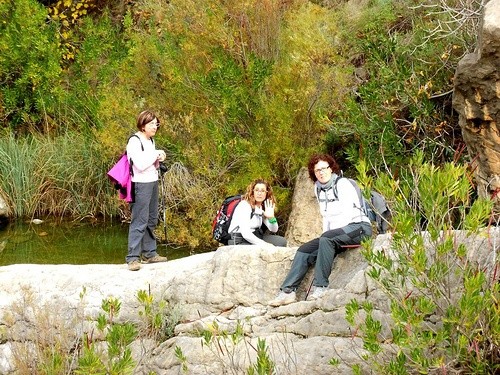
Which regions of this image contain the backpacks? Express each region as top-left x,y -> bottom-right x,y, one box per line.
210,194 -> 242,244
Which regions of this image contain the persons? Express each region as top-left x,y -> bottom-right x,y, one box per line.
227,179 -> 287,247
126,111 -> 167,271
269,154 -> 372,306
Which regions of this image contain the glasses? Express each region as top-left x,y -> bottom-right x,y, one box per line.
314,165 -> 330,174
147,123 -> 161,129
252,188 -> 269,193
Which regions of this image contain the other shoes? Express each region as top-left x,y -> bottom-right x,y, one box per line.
307,285 -> 330,300
128,260 -> 140,271
268,290 -> 297,306
141,253 -> 168,263
